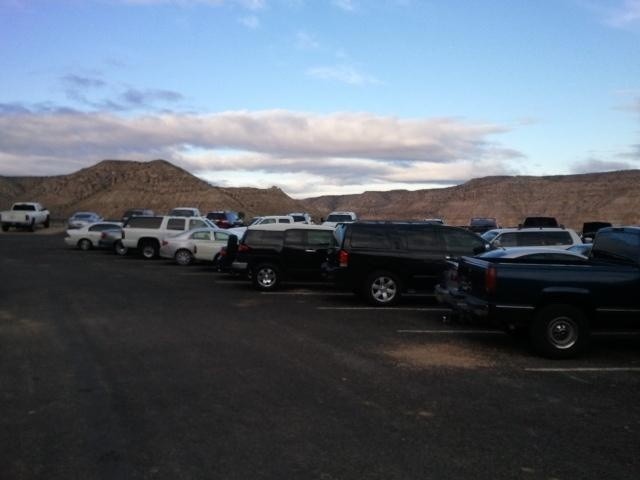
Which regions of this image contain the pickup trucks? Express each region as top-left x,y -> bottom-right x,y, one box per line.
0,202 -> 52,231
